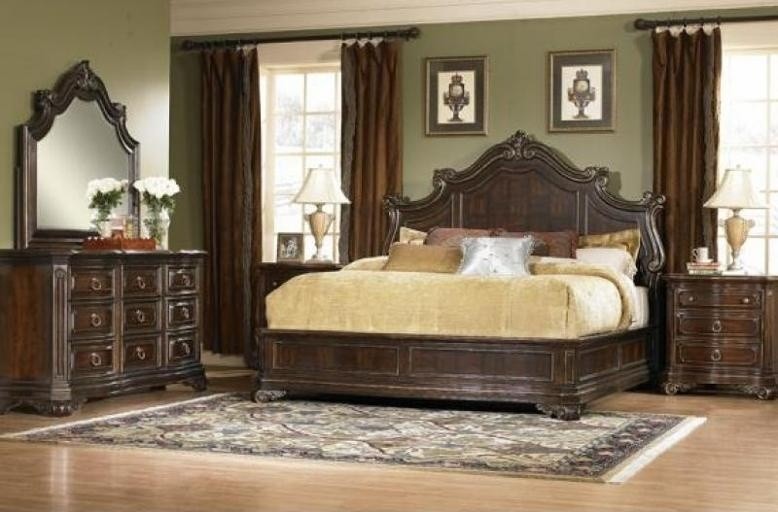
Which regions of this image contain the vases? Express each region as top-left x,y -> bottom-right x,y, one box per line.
140,205 -> 171,251
89,206 -> 114,239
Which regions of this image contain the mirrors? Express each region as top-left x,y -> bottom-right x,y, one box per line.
15,57 -> 141,249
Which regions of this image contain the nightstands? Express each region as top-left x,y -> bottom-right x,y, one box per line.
655,275 -> 777,402
248,265 -> 341,371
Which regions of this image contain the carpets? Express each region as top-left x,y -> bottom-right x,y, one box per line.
0,387 -> 708,484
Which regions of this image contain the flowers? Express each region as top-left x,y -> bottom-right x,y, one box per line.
132,176 -> 181,212
85,177 -> 128,210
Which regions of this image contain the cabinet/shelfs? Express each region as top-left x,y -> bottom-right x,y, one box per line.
0,249 -> 211,417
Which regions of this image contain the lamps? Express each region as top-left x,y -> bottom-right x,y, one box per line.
290,164 -> 352,265
701,164 -> 770,276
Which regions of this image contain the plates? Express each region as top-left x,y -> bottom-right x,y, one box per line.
693,259 -> 714,264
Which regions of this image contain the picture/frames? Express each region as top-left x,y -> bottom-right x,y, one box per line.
546,47 -> 616,136
421,53 -> 489,138
275,232 -> 304,264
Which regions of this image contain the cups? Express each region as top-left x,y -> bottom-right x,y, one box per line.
693,247 -> 708,259
124,214 -> 138,240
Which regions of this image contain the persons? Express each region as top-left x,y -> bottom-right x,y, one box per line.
280,239 -> 289,257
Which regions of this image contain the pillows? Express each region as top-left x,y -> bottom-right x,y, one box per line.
381,220 -> 642,279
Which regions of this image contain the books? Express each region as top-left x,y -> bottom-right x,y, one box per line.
685,262 -> 722,277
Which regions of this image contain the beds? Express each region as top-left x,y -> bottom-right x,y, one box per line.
250,128 -> 665,421
